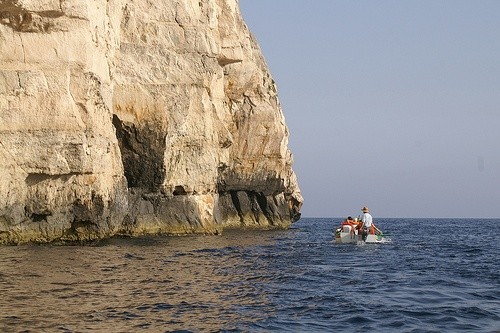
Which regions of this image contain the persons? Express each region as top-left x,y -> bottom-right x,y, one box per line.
359,206 -> 373,240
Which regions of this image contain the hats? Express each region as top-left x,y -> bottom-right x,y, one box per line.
361,206 -> 369,212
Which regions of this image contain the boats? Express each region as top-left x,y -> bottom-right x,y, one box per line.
334,218 -> 388,244
